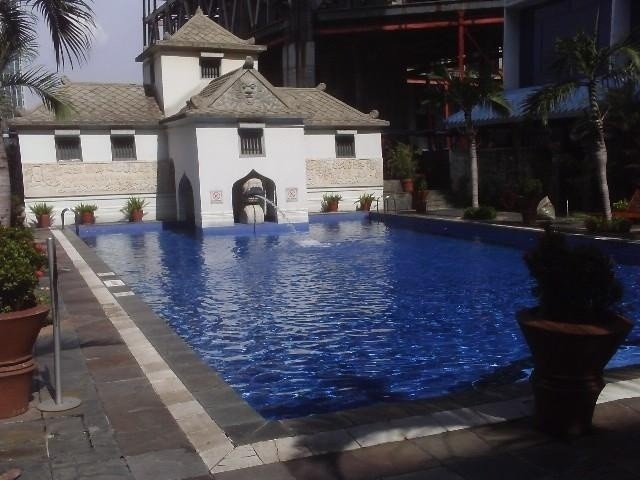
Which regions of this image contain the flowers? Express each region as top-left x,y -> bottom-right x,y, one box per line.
0,225 -> 47,313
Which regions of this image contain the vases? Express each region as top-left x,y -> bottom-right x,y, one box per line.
0,305 -> 50,420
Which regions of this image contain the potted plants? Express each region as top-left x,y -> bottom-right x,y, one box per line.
353,193 -> 378,210
514,224 -> 633,436
120,195 -> 152,221
387,143 -> 422,192
29,201 -> 54,227
416,179 -> 429,200
522,178 -> 543,226
72,202 -> 97,225
322,193 -> 343,211
